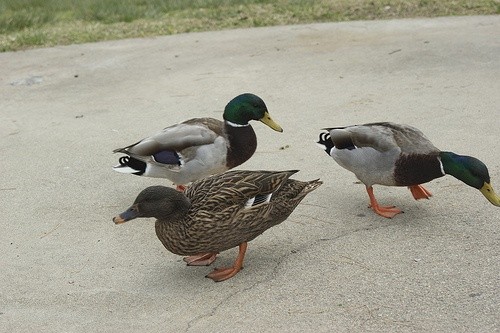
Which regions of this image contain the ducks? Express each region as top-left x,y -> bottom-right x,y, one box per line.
315,121 -> 500,220
112,92 -> 284,193
112,169 -> 324,283
